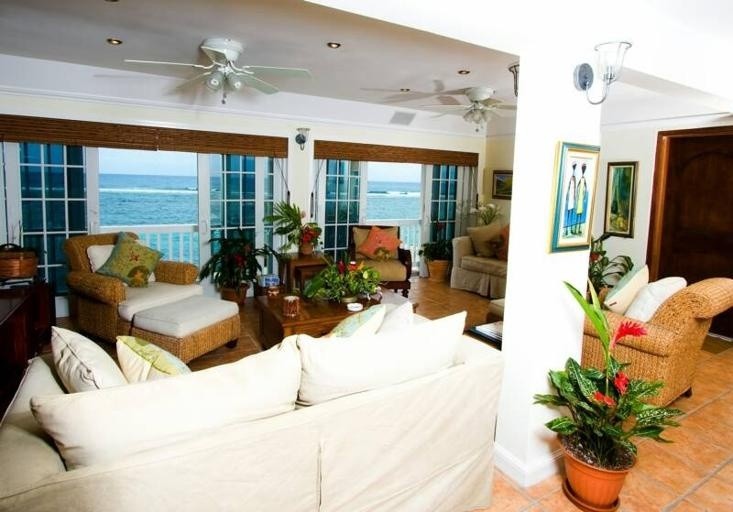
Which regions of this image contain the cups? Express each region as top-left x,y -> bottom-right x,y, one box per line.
268,286 -> 279,300
282,296 -> 300,317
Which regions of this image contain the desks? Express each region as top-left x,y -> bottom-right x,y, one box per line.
286,254 -> 329,293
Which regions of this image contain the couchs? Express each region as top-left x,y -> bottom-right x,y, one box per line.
450,235 -> 509,299
0,282 -> 504,511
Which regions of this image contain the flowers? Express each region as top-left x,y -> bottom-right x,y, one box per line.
303,260 -> 380,300
534,276 -> 686,464
469,203 -> 501,226
271,200 -> 321,244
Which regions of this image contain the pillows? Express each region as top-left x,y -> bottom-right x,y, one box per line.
466,219 -> 509,260
603,264 -> 688,322
352,226 -> 402,265
87,232 -> 164,287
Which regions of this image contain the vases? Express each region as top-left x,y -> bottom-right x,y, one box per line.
557,433 -> 636,512
300,244 -> 313,254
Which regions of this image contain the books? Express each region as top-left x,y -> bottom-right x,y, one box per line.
475,320 -> 502,341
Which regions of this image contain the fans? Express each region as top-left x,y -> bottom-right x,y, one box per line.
421,86 -> 517,119
124,38 -> 313,96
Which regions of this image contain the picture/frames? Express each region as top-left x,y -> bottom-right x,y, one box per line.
492,169 -> 513,201
604,161 -> 638,238
548,142 -> 601,254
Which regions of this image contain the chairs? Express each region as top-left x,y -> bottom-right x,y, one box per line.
582,276 -> 733,409
63,232 -> 201,344
348,225 -> 412,299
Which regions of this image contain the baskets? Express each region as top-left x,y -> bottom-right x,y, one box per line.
0,244 -> 37,277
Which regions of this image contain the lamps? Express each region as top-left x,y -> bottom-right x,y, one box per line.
296,127 -> 310,150
203,65 -> 246,105
575,40 -> 630,105
463,110 -> 493,132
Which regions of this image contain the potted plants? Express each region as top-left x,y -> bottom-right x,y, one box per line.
200,229 -> 270,304
419,239 -> 452,282
